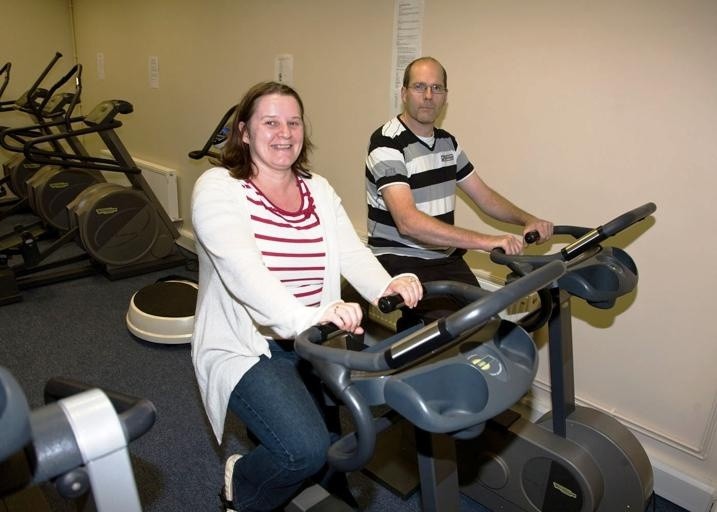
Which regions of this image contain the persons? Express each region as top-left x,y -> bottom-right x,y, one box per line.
189,81 -> 424,512
363,56 -> 554,440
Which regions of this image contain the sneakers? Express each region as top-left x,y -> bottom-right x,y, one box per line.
223,454 -> 244,512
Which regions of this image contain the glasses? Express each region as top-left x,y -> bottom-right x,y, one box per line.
405,82 -> 448,94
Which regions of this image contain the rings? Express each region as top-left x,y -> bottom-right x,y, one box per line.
333,306 -> 340,313
407,276 -> 416,283
